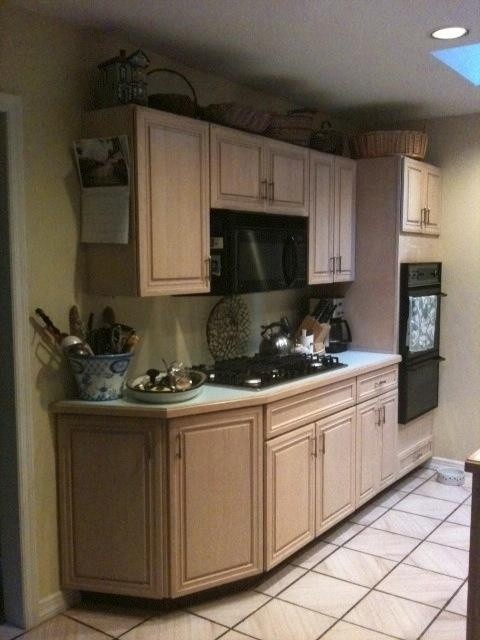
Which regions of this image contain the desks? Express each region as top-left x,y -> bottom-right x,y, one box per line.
465,447 -> 480,640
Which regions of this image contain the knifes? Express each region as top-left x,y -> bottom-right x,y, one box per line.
312,299 -> 338,324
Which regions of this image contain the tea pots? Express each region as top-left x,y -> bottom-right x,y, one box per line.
259,324 -> 294,360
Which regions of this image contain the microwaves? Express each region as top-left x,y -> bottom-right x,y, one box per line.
172,224 -> 308,297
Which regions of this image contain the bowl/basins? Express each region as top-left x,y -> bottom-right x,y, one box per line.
437,467 -> 465,486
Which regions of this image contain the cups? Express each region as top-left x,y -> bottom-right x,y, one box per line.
300,334 -> 314,354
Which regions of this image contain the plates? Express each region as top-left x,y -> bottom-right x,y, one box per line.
126,370 -> 208,403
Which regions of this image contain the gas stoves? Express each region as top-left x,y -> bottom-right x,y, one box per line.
185,353 -> 348,393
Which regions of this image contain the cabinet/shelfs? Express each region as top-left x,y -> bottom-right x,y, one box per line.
56,405 -> 263,611
356,156 -> 441,237
357,363 -> 400,513
262,377 -> 357,583
210,123 -> 309,217
288,149 -> 357,286
81,104 -> 212,298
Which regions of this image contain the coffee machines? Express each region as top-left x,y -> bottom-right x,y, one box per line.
310,293 -> 353,354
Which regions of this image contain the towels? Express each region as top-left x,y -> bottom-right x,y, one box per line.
406,295 -> 437,352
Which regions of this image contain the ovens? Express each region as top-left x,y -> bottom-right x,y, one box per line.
399,263 -> 441,425
